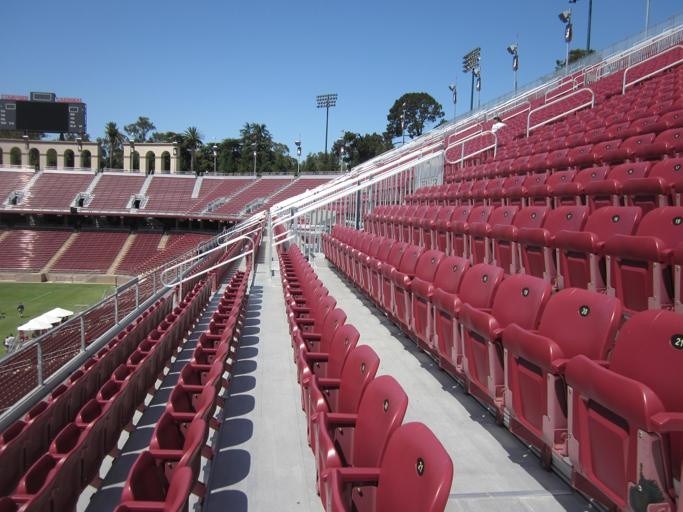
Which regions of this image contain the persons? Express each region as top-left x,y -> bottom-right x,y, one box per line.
3,334 -> 17,352
16,302 -> 25,317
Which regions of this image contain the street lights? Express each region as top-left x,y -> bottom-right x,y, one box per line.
461,47 -> 481,109
447,81 -> 456,122
472,66 -> 482,109
249,142 -> 258,176
558,8 -> 573,77
507,38 -> 520,96
336,138 -> 345,174
212,145 -> 216,174
397,100 -> 406,144
294,141 -> 302,174
315,92 -> 337,161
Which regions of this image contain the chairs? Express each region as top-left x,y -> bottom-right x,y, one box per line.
2,211 -> 263,512
269,37 -> 683,512
1,170 -> 348,274
